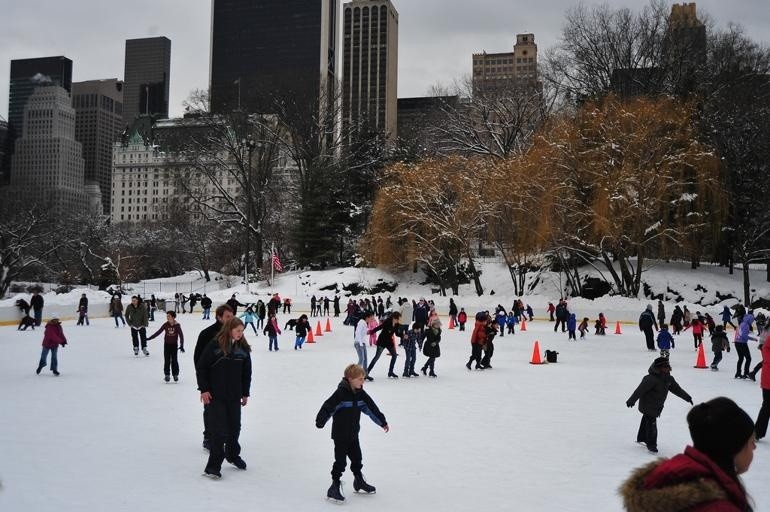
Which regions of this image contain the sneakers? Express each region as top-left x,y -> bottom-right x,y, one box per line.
749,371 -> 756,382
429,372 -> 438,376
37,366 -> 42,374
475,364 -> 483,369
53,370 -> 60,374
164,375 -> 170,381
735,372 -> 742,377
402,373 -> 410,377
743,372 -> 749,378
205,465 -> 222,477
269,348 -> 272,351
364,376 -> 374,381
299,344 -> 301,349
410,371 -> 419,376
637,439 -> 646,444
646,445 -> 658,453
294,345 -> 297,350
143,347 -> 150,355
327,485 -> 345,501
421,367 -> 427,375
173,375 -> 178,381
274,347 -> 280,350
133,346 -> 139,355
711,366 -> 717,369
711,362 -> 716,365
388,372 -> 398,377
466,363 -> 471,370
226,455 -> 246,469
353,478 -> 375,492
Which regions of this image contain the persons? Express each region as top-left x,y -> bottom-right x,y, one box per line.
343,295 -> 442,381
146,311 -> 185,382
36,315 -> 67,375
203,317 -> 252,479
310,295 -> 341,317
466,299 -> 534,370
175,293 -> 212,319
753,333 -> 770,441
77,294 -> 89,325
16,293 -> 44,330
109,294 -> 157,357
626,357 -> 693,452
547,299 -> 607,341
619,396 -> 758,512
316,364 -> 389,502
639,300 -> 769,381
227,292 -> 311,351
449,298 -> 468,331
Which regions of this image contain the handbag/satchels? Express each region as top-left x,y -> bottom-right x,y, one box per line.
544,350 -> 559,363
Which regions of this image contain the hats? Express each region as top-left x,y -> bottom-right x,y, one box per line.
647,304 -> 652,310
49,313 -> 60,321
687,397 -> 754,456
431,319 -> 440,325
655,357 -> 669,366
691,315 -> 698,319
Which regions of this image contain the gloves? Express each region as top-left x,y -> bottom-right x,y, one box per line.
431,342 -> 436,347
146,338 -> 150,341
178,346 -> 185,352
626,400 -> 635,408
688,399 -> 693,405
727,347 -> 730,352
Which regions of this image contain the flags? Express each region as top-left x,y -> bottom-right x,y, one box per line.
273,249 -> 283,272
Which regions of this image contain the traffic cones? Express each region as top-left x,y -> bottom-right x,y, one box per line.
326,318 -> 331,332
448,318 -> 455,329
306,327 -> 316,344
693,341 -> 711,370
520,320 -> 527,332
529,340 -> 544,365
614,321 -> 623,334
313,320 -> 324,336
